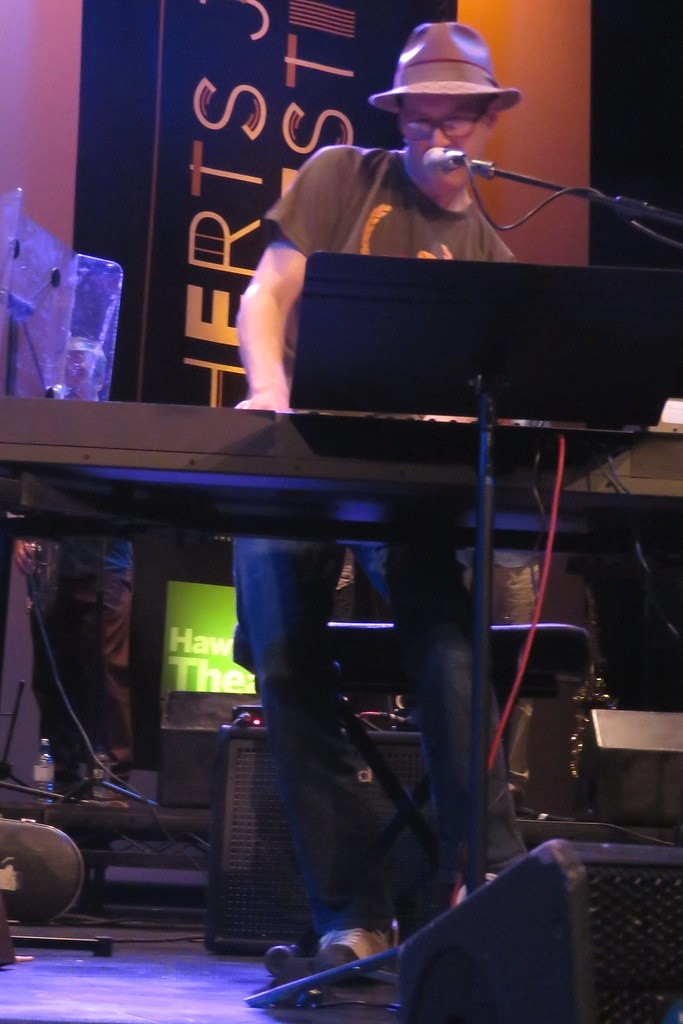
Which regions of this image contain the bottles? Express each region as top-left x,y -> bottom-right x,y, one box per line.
32,739 -> 56,804
92,754 -> 112,798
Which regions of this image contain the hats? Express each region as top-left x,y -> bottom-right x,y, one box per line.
368,23 -> 522,113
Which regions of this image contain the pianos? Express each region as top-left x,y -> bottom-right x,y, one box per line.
0,396 -> 683,966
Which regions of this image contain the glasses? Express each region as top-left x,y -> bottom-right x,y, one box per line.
400,102 -> 490,138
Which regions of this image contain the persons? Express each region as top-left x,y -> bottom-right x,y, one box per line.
13,526 -> 132,798
235,21 -> 532,983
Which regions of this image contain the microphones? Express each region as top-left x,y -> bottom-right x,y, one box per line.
422,146 -> 467,174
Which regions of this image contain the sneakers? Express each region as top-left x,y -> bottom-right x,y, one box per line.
320,928 -> 389,956
453,874 -> 497,908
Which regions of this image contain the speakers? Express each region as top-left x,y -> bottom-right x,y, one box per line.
204,723 -> 446,955
157,690 -> 260,808
508,695 -> 683,826
397,837 -> 683,1024
